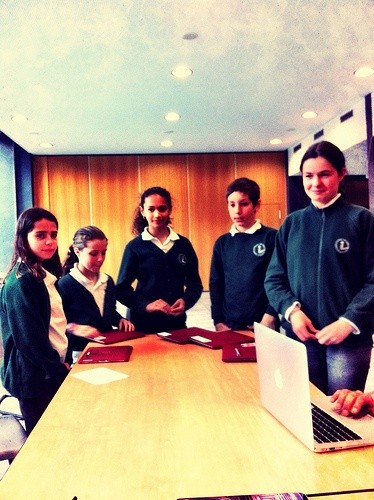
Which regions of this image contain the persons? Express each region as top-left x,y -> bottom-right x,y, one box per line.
264,141 -> 374,395
59,225 -> 135,363
116,187 -> 203,334
210,178 -> 281,335
0,208 -> 73,436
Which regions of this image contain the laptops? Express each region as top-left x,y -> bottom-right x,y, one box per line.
253,322 -> 374,452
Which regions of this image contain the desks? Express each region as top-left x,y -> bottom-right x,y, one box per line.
0,334 -> 374,500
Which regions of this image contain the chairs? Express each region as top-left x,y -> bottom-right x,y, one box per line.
0,415 -> 28,465
